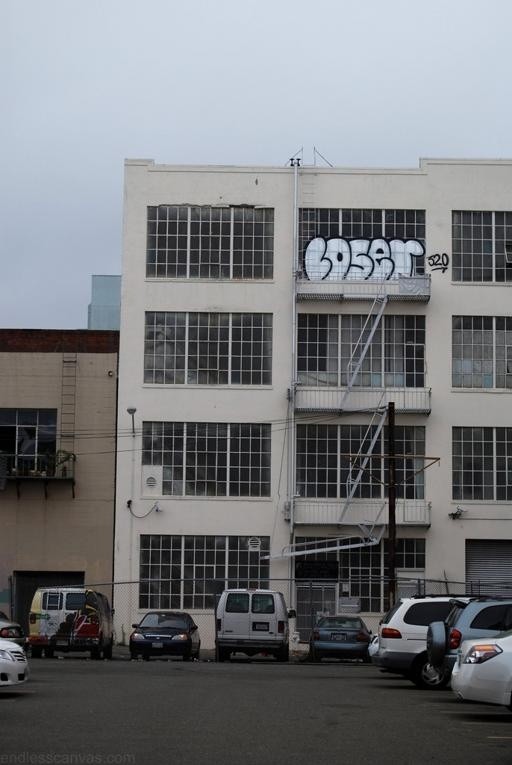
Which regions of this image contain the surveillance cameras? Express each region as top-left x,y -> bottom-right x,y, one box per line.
456,505 -> 468,512
